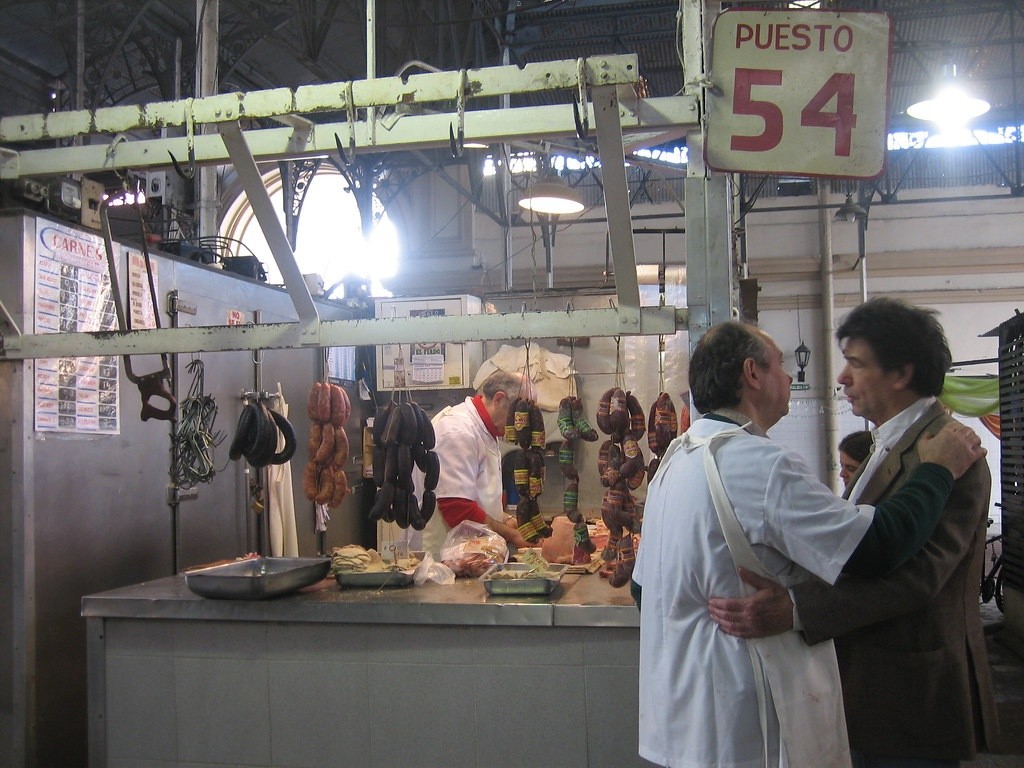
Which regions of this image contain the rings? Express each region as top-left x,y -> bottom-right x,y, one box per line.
730,620 -> 736,632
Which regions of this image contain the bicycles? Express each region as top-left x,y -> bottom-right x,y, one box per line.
978,501 -> 1005,615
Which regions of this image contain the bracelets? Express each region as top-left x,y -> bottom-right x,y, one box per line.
502,514 -> 517,526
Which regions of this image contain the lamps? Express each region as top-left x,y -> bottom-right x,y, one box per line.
906,57 -> 991,121
795,341 -> 811,381
517,155 -> 584,214
833,179 -> 867,222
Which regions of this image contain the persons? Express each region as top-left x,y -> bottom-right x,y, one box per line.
405,371 -> 544,560
629,297 -> 991,768
